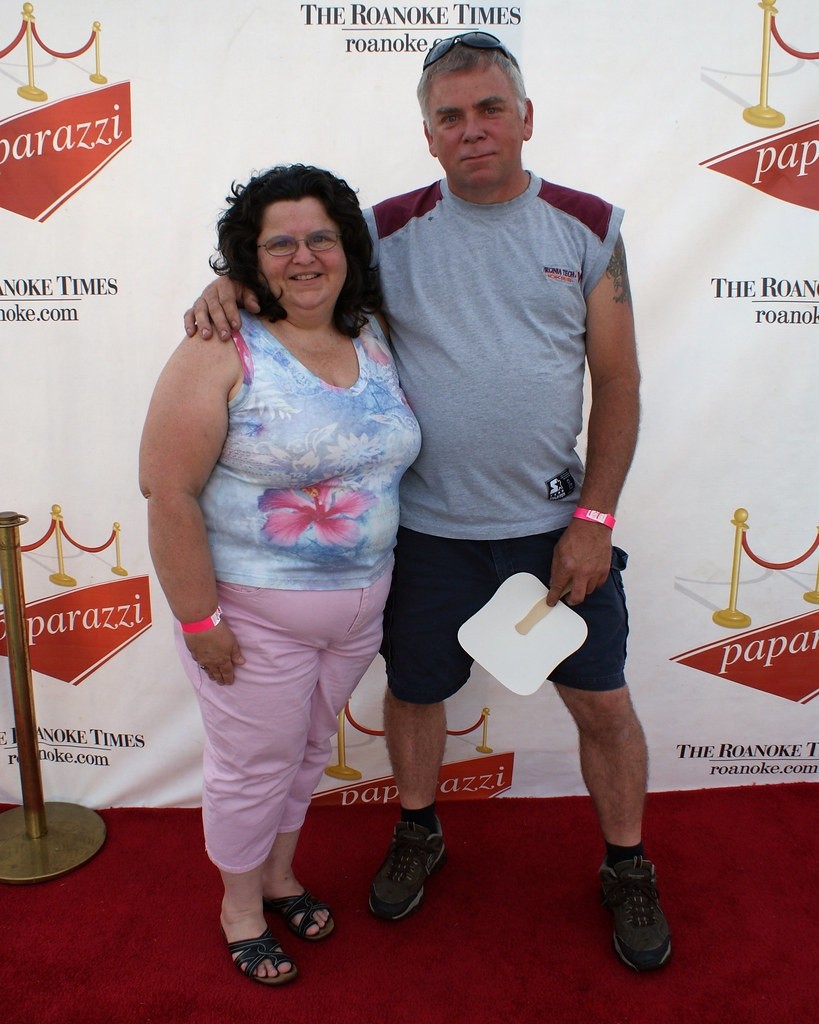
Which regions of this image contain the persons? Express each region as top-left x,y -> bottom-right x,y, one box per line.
183,33 -> 672,974
138,164 -> 424,984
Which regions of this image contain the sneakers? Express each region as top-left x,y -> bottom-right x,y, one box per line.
365,813 -> 448,923
598,854 -> 672,972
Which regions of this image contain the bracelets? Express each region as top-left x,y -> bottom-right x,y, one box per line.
181,606 -> 223,634
572,507 -> 616,529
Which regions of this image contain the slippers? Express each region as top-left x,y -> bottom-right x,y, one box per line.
218,917 -> 299,986
259,887 -> 335,940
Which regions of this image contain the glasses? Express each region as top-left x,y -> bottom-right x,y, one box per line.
423,31 -> 509,71
256,230 -> 343,257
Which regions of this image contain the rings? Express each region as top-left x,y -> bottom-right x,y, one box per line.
201,666 -> 205,669
